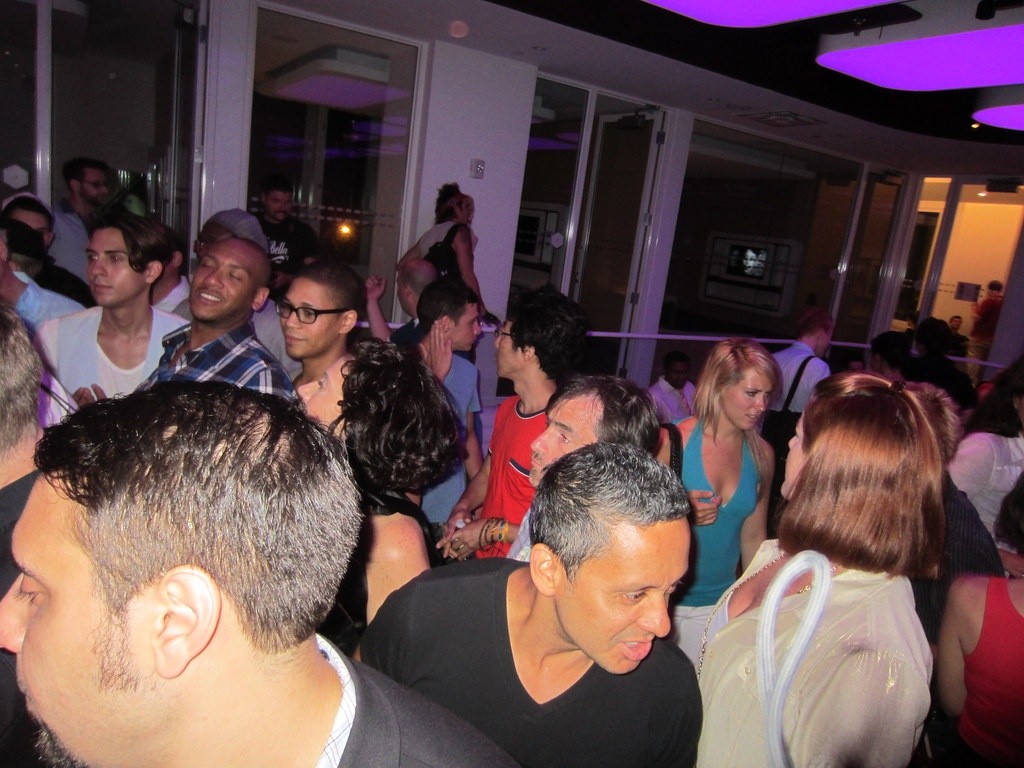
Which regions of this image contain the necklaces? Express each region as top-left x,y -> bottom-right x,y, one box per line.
797,561 -> 839,593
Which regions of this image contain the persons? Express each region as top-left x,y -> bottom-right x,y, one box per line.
0,157 -> 1024,651
655,339 -> 780,667
936,471 -> 1024,768
0,379 -> 523,768
697,369 -> 944,768
352,442 -> 704,768
0,303 -> 46,752
71,237 -> 305,408
296,336 -> 465,662
506,375 -> 661,562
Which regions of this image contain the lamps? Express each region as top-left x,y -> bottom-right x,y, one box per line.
256,42 -> 393,109
0,0 -> 91,47
644,0 -> 1024,132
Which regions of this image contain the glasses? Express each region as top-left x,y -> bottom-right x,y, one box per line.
276,301 -> 348,323
494,322 -> 517,339
196,241 -> 207,250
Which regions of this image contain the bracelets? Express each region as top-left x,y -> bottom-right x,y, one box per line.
479,518 -> 508,552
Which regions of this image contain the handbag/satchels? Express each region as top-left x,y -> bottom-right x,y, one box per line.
424,222 -> 465,286
761,355 -> 818,455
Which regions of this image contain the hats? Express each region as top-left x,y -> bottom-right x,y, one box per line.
208,207 -> 267,254
